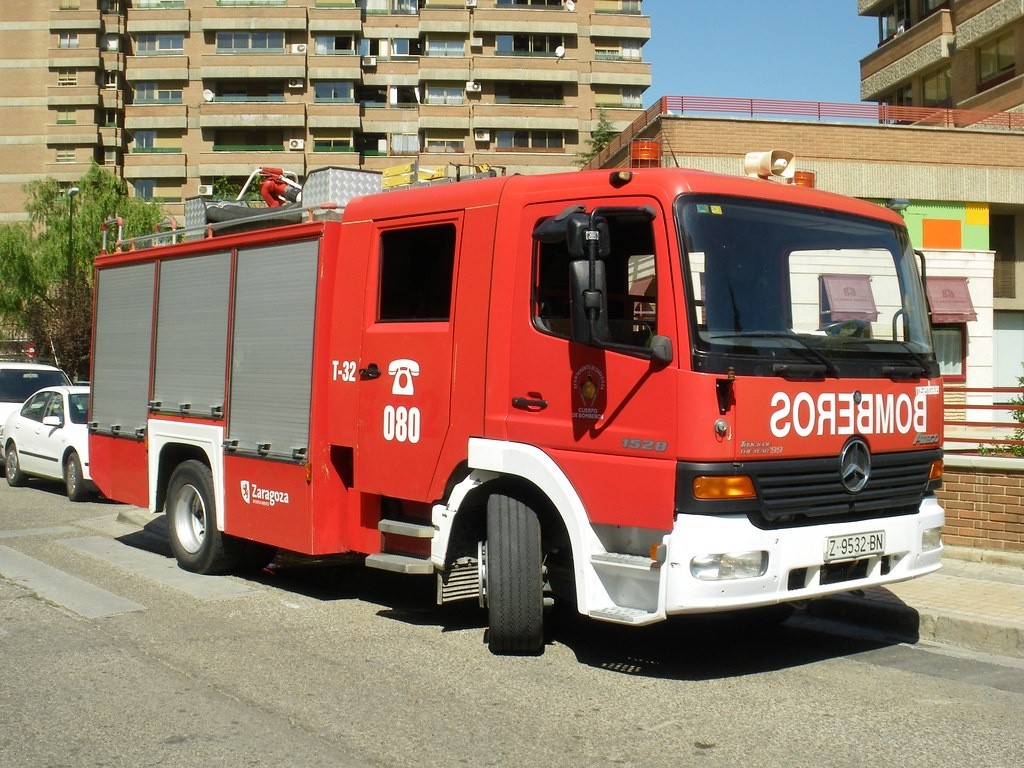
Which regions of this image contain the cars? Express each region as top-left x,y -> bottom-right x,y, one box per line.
0,385 -> 106,502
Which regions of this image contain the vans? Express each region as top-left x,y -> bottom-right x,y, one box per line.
0,362 -> 84,478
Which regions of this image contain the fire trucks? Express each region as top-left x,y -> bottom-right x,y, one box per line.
88,149 -> 948,655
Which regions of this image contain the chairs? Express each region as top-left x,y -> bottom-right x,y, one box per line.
35,405 -> 56,423
71,404 -> 80,424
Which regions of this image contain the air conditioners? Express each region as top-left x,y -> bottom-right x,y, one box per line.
362,56 -> 377,66
466,82 -> 482,92
288,79 -> 304,88
291,44 -> 307,55
471,38 -> 483,46
198,185 -> 213,195
475,129 -> 490,142
896,19 -> 910,37
289,139 -> 304,150
466,0 -> 477,7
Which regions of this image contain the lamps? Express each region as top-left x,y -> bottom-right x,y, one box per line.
886,199 -> 910,210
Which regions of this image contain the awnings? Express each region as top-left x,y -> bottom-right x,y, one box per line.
921,276 -> 978,323
822,273 -> 880,322
